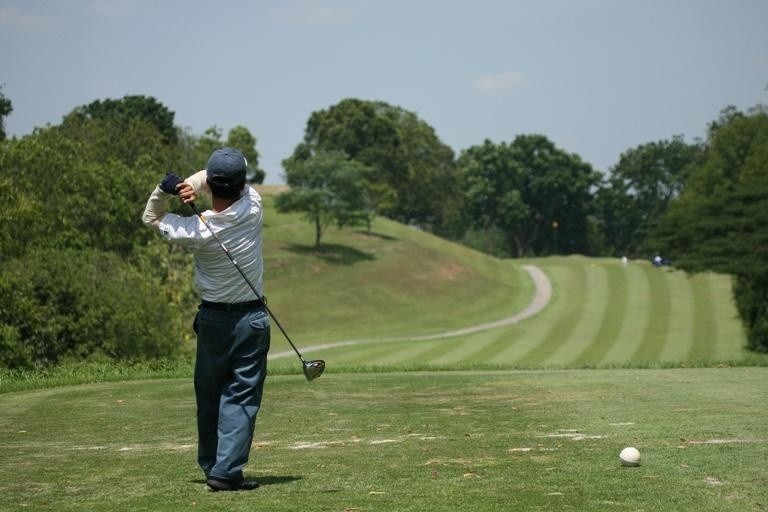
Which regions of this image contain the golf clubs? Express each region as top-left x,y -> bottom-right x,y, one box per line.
166,174 -> 326,382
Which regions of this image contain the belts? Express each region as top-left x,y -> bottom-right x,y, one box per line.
202,296 -> 267,315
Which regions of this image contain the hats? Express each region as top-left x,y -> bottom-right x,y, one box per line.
206,147 -> 248,194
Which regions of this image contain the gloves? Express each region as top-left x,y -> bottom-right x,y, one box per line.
159,173 -> 184,194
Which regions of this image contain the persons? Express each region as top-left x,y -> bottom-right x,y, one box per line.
142,147 -> 271,489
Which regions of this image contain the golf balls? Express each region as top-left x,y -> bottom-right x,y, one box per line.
619,445 -> 640,465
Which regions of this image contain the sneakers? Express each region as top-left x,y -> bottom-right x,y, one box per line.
206,476 -> 260,490
